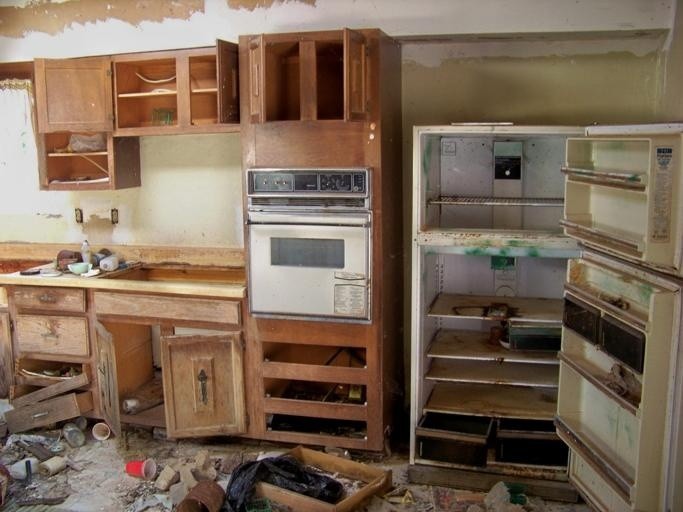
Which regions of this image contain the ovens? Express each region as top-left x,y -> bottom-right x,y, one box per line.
246,167 -> 374,323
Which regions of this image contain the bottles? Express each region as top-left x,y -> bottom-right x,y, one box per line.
82,239 -> 91,264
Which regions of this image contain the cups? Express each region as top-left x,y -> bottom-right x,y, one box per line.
125,458 -> 157,480
92,422 -> 112,441
62,422 -> 86,447
122,399 -> 139,412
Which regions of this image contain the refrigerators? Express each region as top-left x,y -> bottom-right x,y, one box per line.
410,122 -> 682,510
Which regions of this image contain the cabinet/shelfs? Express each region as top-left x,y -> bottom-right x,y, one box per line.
31,57 -> 141,192
87,291 -> 246,439
6,290 -> 94,420
237,26 -> 401,125
111,39 -> 237,136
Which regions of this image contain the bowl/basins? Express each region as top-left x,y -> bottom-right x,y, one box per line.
67,262 -> 93,275
